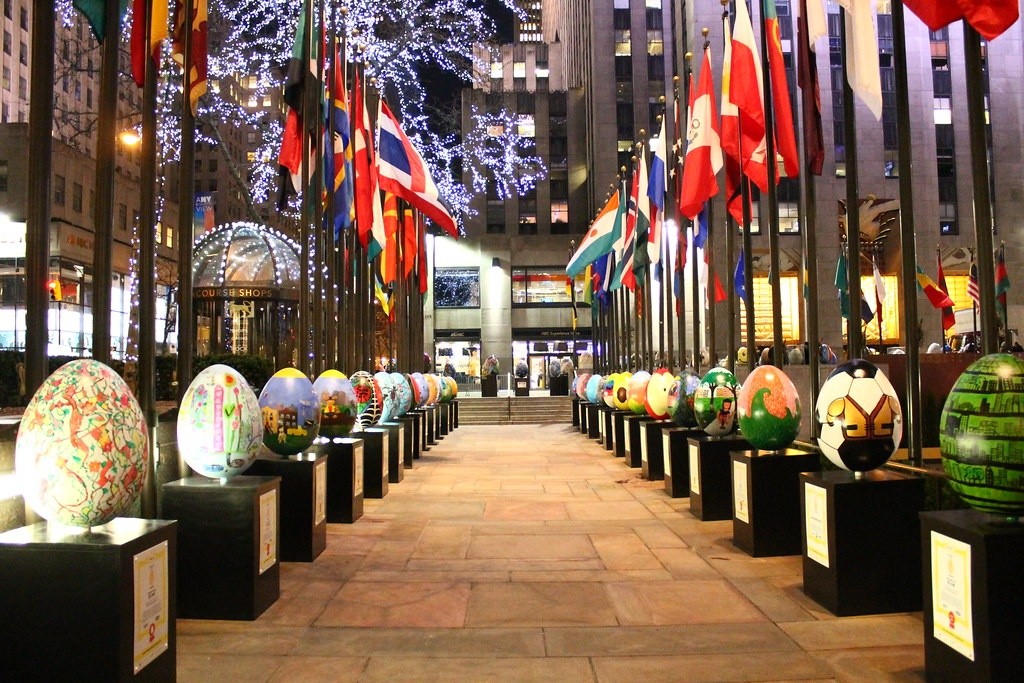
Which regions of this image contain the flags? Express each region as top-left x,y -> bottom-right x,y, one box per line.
993,244 -> 1011,332
130,0 -> 168,88
917,265 -> 956,309
806,0 -> 825,53
834,251 -> 874,325
803,260 -> 809,304
713,267 -> 727,302
720,17 -> 754,228
937,254 -> 956,331
170,0 -> 208,118
72,1 -> 105,45
967,261 -> 982,317
734,246 -> 746,302
762,0 -> 798,178
903,0 -> 1020,42
872,264 -> 887,328
680,45 -> 723,222
276,0 -> 459,324
683,73 -> 708,250
729,0 -> 781,196
798,0 -> 825,176
564,98 -> 688,330
835,0 -> 882,122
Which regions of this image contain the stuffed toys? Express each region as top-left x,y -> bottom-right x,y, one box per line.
598,327 -> 1023,366
